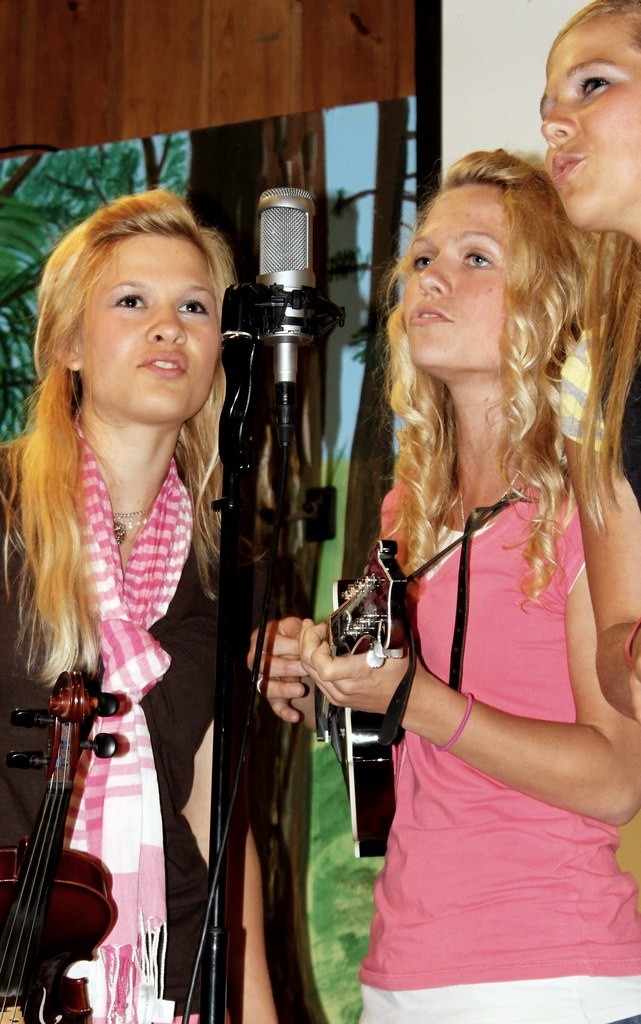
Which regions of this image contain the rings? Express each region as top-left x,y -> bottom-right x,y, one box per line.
255,677 -> 265,694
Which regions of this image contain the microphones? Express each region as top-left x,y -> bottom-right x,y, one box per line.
257,188 -> 317,449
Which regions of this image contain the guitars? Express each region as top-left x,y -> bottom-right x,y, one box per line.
323,541 -> 407,858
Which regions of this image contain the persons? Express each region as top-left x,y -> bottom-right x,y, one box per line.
538,0 -> 641,731
0,188 -> 314,1024
246,146 -> 641,1024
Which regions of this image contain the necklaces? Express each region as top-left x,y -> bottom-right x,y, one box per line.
108,506 -> 154,548
455,470 -> 523,534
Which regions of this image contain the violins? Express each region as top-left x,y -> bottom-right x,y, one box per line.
0,669 -> 118,1024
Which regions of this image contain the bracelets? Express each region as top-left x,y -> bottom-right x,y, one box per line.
433,691 -> 476,753
